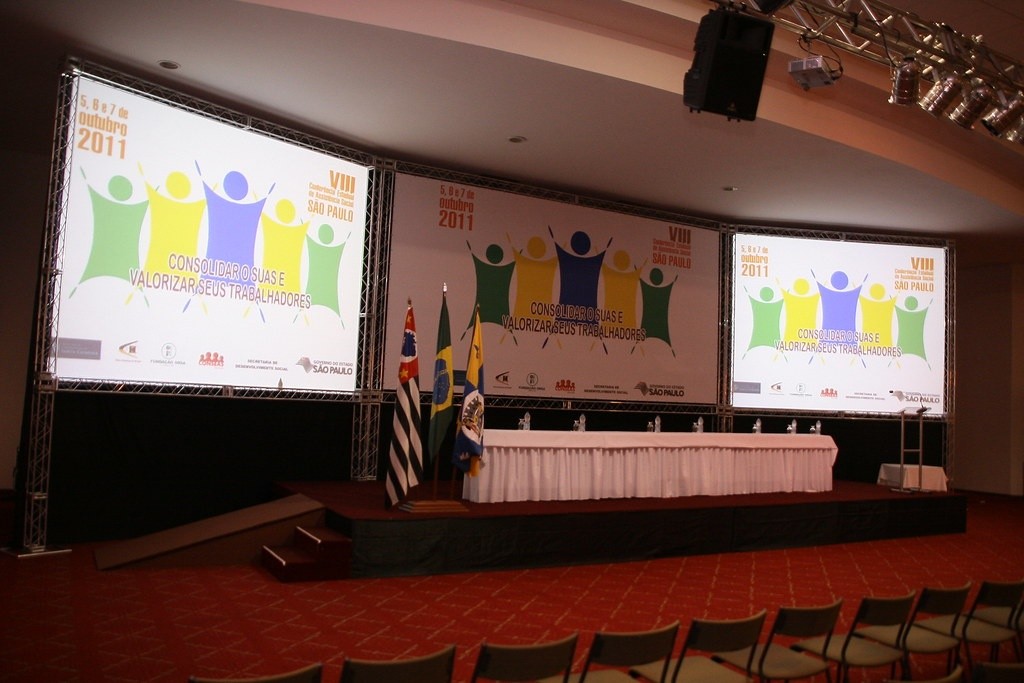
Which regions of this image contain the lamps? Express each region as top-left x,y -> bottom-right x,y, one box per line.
888,54 -> 1024,145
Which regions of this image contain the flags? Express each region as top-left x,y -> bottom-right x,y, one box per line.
425,294 -> 456,479
453,310 -> 485,479
383,307 -> 425,510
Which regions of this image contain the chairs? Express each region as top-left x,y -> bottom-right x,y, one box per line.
190,579 -> 1024,683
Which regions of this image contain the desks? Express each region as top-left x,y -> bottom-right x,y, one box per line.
462,429 -> 839,503
877,464 -> 949,492
